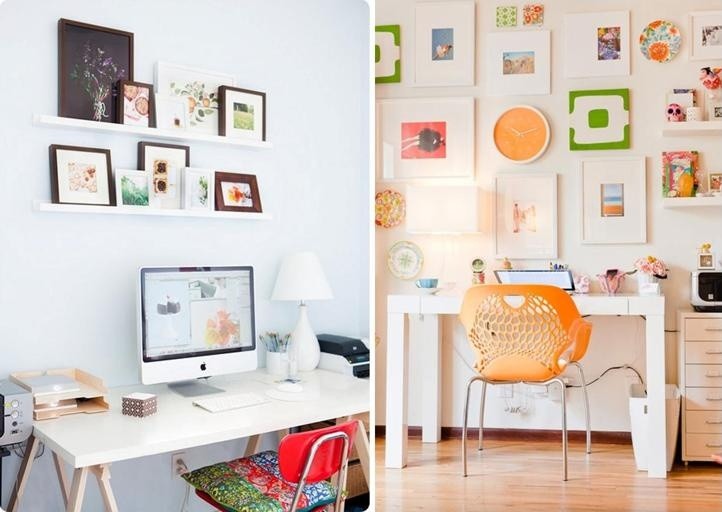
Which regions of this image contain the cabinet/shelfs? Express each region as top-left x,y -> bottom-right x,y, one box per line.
676,309 -> 722,466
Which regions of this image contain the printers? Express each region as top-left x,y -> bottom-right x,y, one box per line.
316,332 -> 370,378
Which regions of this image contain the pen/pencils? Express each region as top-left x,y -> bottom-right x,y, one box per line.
259,333 -> 291,352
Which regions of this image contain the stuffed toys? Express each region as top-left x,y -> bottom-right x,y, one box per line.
666,102 -> 687,123
698,65 -> 722,90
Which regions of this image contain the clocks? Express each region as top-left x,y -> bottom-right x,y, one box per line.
492,104 -> 551,164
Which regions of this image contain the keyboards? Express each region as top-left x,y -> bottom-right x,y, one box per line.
192,393 -> 270,412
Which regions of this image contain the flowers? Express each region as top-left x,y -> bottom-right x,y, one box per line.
633,256 -> 671,280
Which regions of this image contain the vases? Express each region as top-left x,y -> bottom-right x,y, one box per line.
638,271 -> 657,292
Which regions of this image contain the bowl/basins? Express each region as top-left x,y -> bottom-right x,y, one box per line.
594,273 -> 625,294
415,278 -> 437,288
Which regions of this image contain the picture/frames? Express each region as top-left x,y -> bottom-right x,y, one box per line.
376,2 -> 476,185
686,11 -> 722,63
492,156 -> 647,260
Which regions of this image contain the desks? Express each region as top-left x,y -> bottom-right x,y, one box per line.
385,292 -> 666,478
2,344 -> 370,512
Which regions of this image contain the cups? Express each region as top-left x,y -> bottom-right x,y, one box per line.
471,270 -> 485,286
267,350 -> 288,373
686,106 -> 705,122
280,344 -> 300,382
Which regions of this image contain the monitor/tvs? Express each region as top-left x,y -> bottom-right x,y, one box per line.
135,264 -> 258,398
492,269 -> 576,291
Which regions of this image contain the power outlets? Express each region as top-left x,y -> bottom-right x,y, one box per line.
496,378 -> 515,398
170,454 -> 187,481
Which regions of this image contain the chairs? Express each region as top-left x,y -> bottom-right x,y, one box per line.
458,283 -> 593,480
195,420 -> 361,512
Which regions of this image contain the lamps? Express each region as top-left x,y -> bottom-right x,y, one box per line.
269,251 -> 334,374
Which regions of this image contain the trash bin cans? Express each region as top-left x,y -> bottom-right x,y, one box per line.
627,383 -> 682,472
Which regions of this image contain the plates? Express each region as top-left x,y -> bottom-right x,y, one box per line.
415,288 -> 443,294
385,240 -> 425,280
639,20 -> 681,64
374,189 -> 407,231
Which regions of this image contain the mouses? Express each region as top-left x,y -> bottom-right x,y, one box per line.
275,378 -> 303,393
